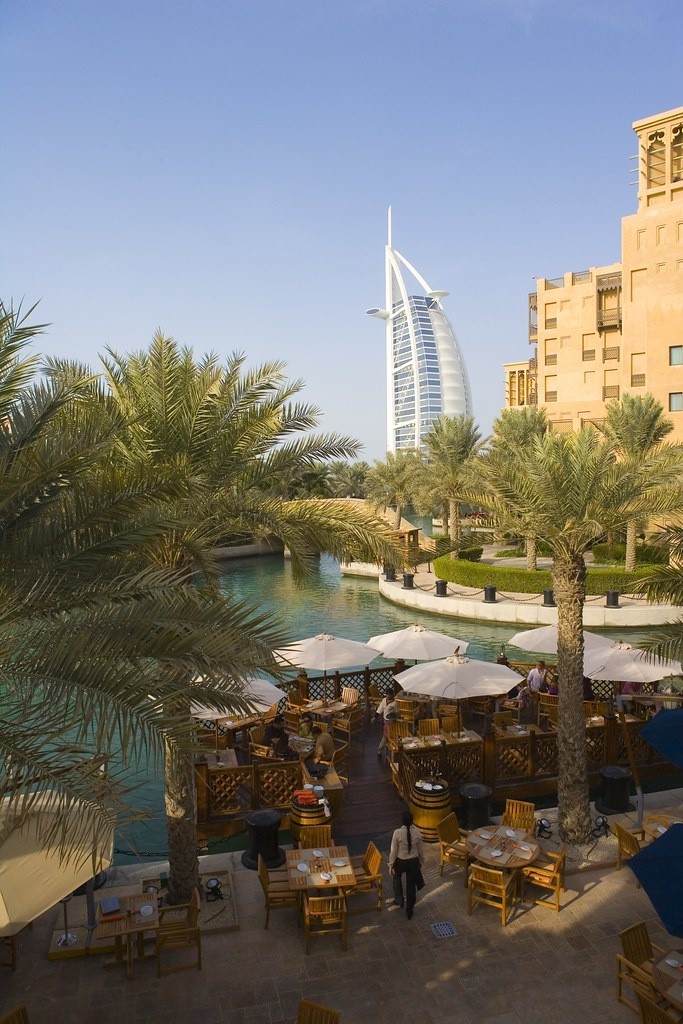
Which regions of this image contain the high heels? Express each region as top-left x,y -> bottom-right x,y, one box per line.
407,910 -> 413,920
393,900 -> 404,908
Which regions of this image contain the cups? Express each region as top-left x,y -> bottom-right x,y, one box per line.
313,786 -> 324,797
303,784 -> 313,790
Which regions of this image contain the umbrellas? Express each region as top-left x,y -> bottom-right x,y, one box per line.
367,623 -> 469,666
508,624 -> 616,654
271,632 -> 384,707
583,644 -> 683,683
640,708 -> 683,768
0,788 -> 118,938
392,651 -> 526,739
148,673 -> 288,750
625,823 -> 683,940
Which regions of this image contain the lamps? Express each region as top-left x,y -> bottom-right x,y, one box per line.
141,886 -> 162,909
205,877 -> 224,902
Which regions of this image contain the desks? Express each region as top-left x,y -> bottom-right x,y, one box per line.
614,693 -> 683,718
643,815 -> 683,840
392,729 -> 481,758
274,734 -> 315,765
96,894 -> 159,979
652,948 -> 683,1012
494,723 -> 542,739
286,846 -> 357,912
466,826 -> 540,873
212,716 -> 262,747
303,697 -> 352,724
584,712 -> 640,734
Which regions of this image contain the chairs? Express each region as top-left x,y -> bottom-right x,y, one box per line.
258,854 -> 304,930
635,988 -> 683,1024
297,825 -> 335,850
191,675 -> 624,759
496,798 -> 537,841
517,843 -> 567,912
613,820 -> 654,889
467,857 -> 518,928
616,923 -> 667,1016
272,998 -> 341,1024
342,841 -> 383,912
0,1002 -> 31,1024
436,812 -> 473,889
156,887 -> 203,977
300,887 -> 348,953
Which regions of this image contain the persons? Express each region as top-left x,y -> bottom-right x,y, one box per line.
526,661 -> 548,697
621,681 -> 643,714
309,726 -> 335,765
429,695 -> 442,719
264,717 -> 285,747
548,674 -> 558,695
653,685 -> 664,696
299,716 -> 317,740
387,811 -> 424,919
371,688 -> 399,761
